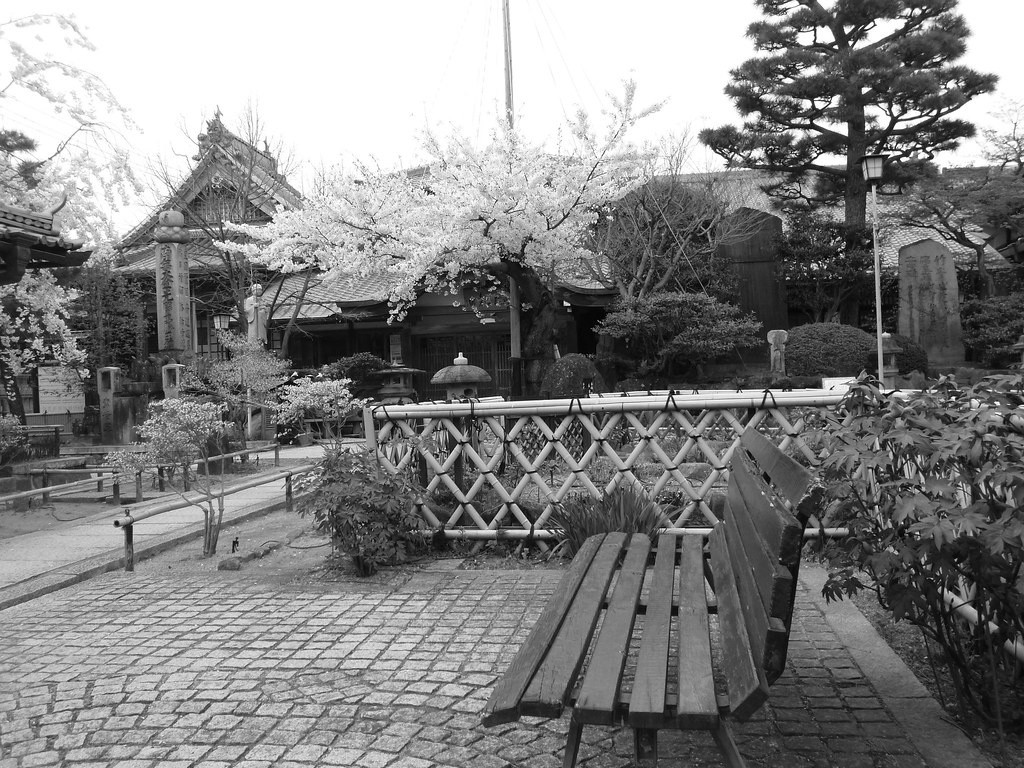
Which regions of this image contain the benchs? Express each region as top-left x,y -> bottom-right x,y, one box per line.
480,427 -> 825,768
